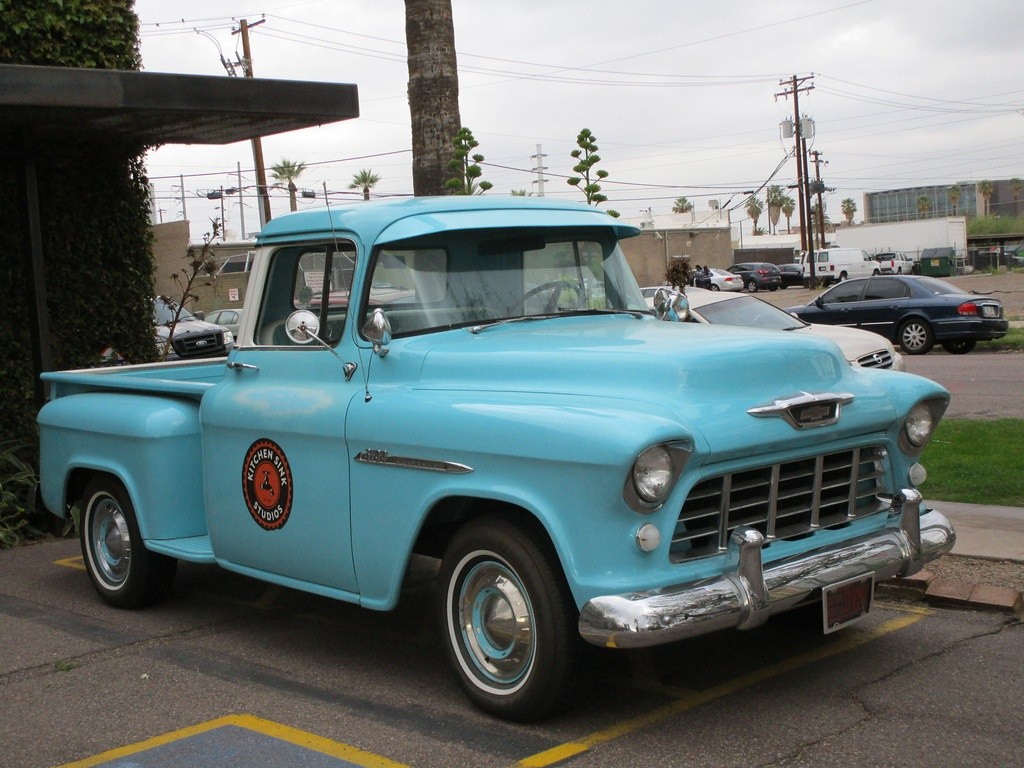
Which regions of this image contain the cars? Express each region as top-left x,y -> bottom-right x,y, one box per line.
753,274 -> 1009,355
873,252 -> 915,274
635,287 -> 712,313
776,264 -> 820,289
148,296 -> 236,362
651,291 -> 906,374
665,268 -> 745,293
200,309 -> 242,342
725,262 -> 782,293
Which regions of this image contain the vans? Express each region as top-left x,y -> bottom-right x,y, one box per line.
800,248 -> 883,287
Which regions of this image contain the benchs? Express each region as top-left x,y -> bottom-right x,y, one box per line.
261,306 -> 501,345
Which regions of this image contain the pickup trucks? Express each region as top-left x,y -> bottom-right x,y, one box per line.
35,195 -> 956,723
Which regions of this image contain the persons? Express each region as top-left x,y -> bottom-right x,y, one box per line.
693,265 -> 705,288
702,266 -> 713,290
